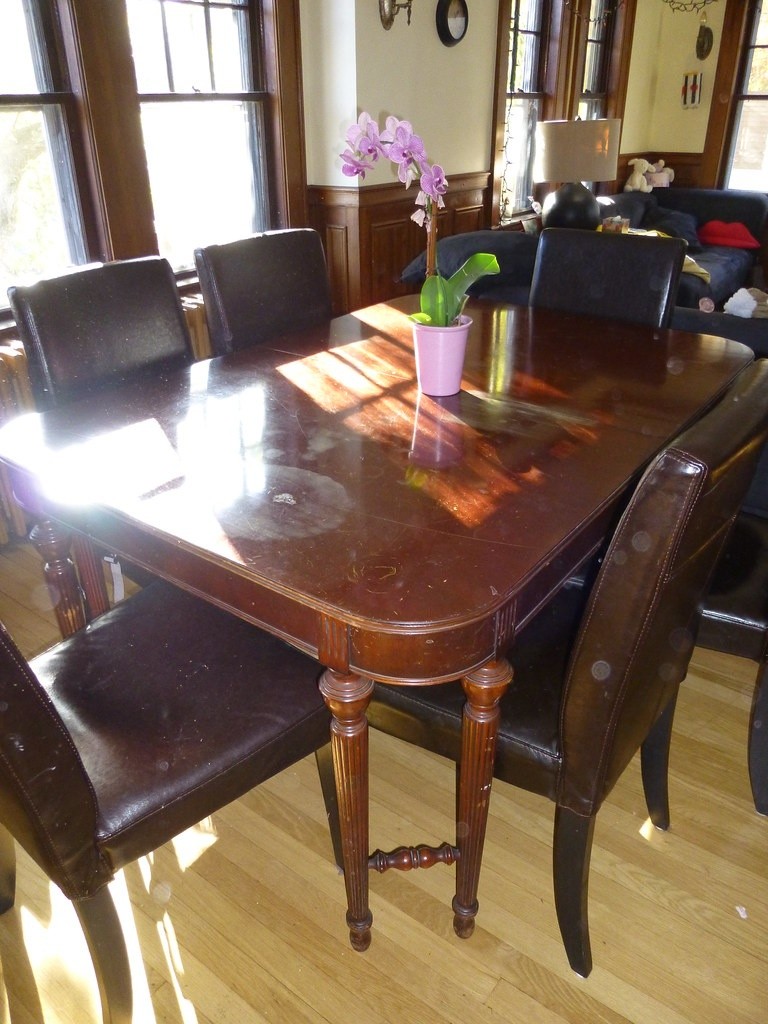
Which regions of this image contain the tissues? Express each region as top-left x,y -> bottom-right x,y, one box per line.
602,215 -> 630,233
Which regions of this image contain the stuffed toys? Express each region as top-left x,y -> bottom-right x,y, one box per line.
623,158 -> 674,193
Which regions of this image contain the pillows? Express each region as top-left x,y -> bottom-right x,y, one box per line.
398,230 -> 540,303
698,219 -> 761,250
642,203 -> 703,252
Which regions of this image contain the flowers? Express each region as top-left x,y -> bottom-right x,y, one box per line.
324,112 -> 497,324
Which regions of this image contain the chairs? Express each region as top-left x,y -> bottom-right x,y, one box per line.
193,228 -> 335,360
333,357 -> 766,978
0,574 -> 353,1021
530,230 -> 689,332
7,253 -> 194,426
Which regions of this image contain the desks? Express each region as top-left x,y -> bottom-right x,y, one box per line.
1,295 -> 756,952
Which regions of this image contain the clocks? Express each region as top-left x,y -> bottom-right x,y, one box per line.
436,0 -> 469,45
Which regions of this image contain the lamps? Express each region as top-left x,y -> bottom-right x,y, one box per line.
529,116 -> 624,226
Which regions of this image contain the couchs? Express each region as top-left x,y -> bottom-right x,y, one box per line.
400,188 -> 767,316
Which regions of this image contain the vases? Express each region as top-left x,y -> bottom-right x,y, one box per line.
412,313 -> 469,393
